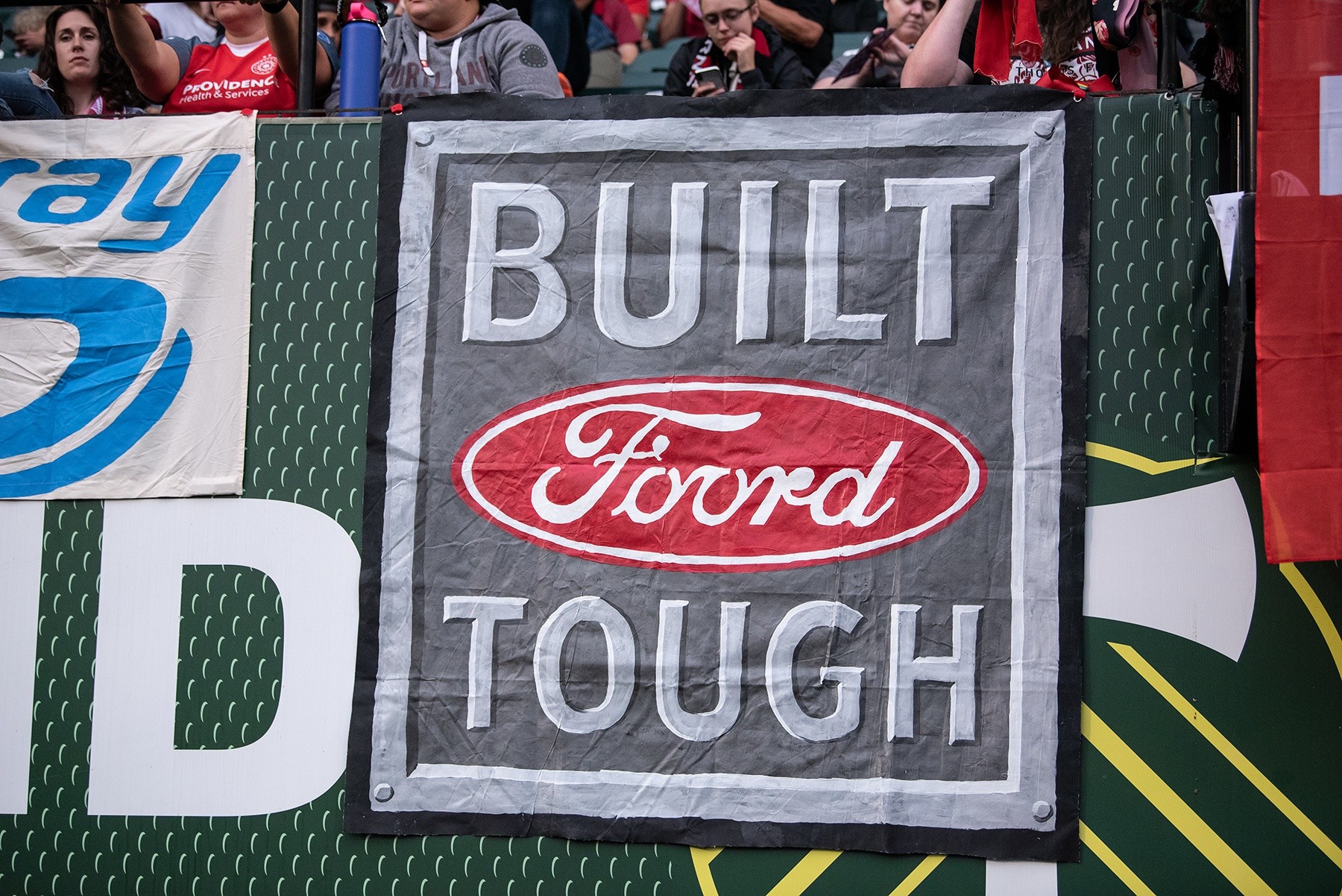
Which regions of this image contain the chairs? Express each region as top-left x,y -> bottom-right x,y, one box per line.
620,32 -> 870,88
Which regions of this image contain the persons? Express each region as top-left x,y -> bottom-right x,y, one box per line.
810,0 -> 975,91
661,1 -> 817,96
0,0 -> 1254,111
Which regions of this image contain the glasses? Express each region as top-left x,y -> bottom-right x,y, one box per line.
700,0 -> 755,27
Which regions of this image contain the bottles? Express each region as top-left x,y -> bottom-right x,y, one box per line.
338,2 -> 387,117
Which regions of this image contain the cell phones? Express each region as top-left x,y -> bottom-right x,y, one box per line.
694,66 -> 728,96
